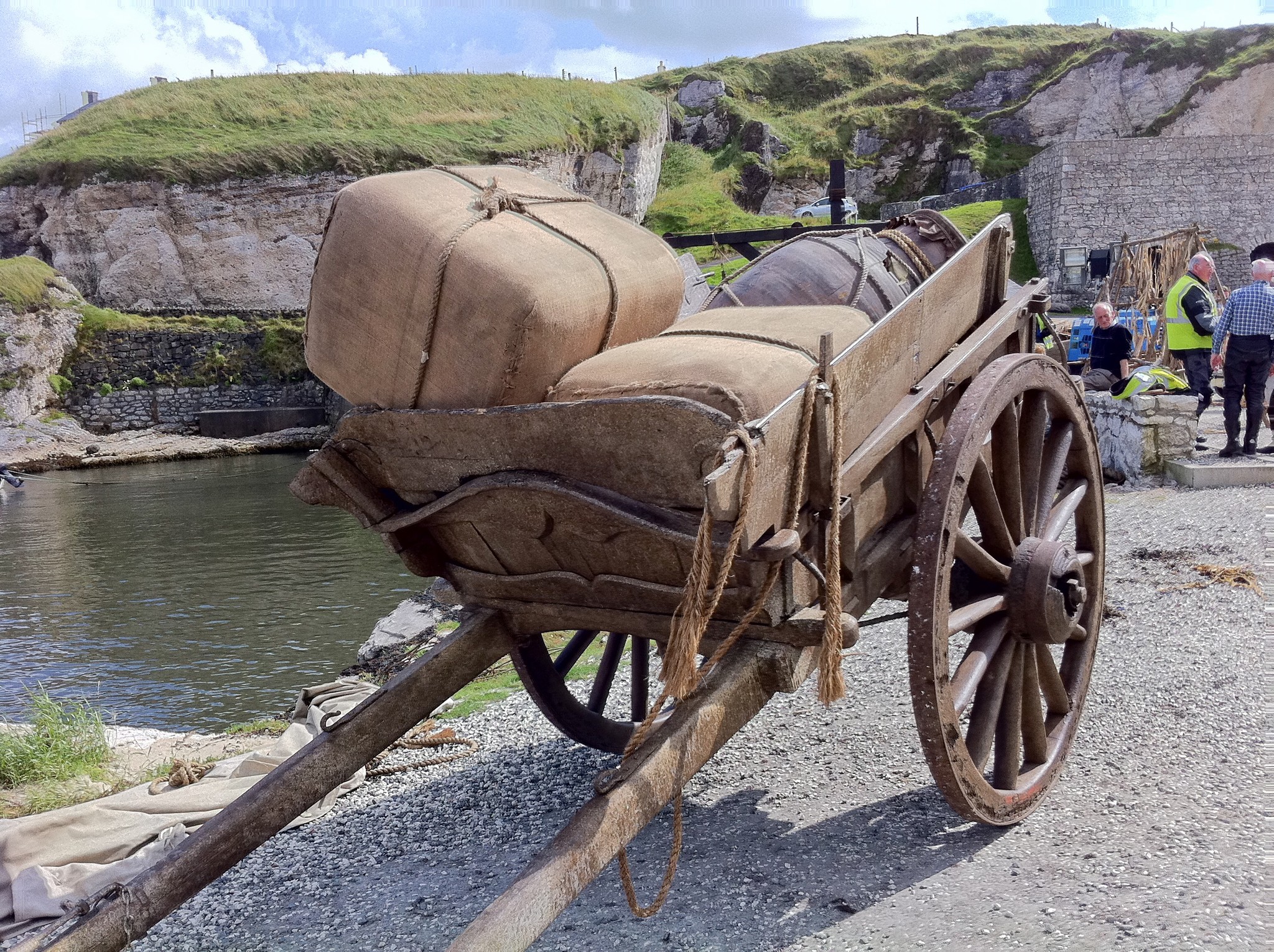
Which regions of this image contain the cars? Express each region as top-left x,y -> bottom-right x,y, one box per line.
790,197 -> 857,219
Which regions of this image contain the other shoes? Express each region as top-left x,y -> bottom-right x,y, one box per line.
1243,436 -> 1257,455
1218,437 -> 1241,456
1255,447 -> 1274,453
1196,434 -> 1208,450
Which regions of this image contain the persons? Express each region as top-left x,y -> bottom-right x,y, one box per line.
1081,300 -> 1134,393
1216,242 -> 1274,455
1165,248 -> 1222,451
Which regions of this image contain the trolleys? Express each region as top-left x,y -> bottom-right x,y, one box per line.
17,163 -> 1108,950
